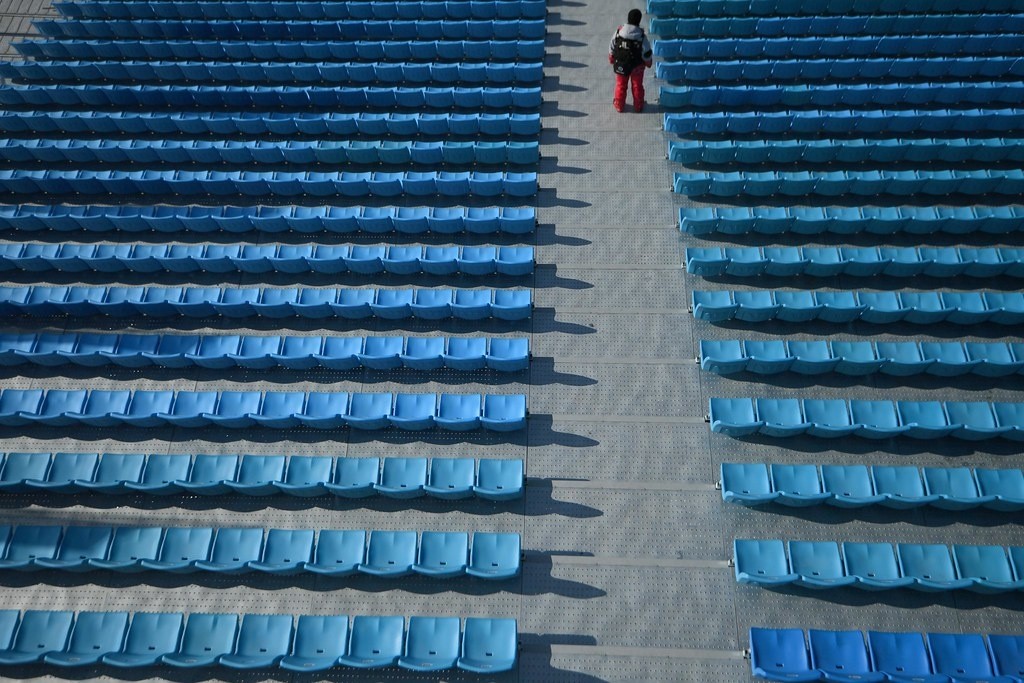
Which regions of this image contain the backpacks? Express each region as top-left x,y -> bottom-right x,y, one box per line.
613,28 -> 645,76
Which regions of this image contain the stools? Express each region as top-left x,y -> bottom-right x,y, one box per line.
0,0 -> 1024,683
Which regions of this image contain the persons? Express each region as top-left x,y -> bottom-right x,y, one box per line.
607,9 -> 654,111
